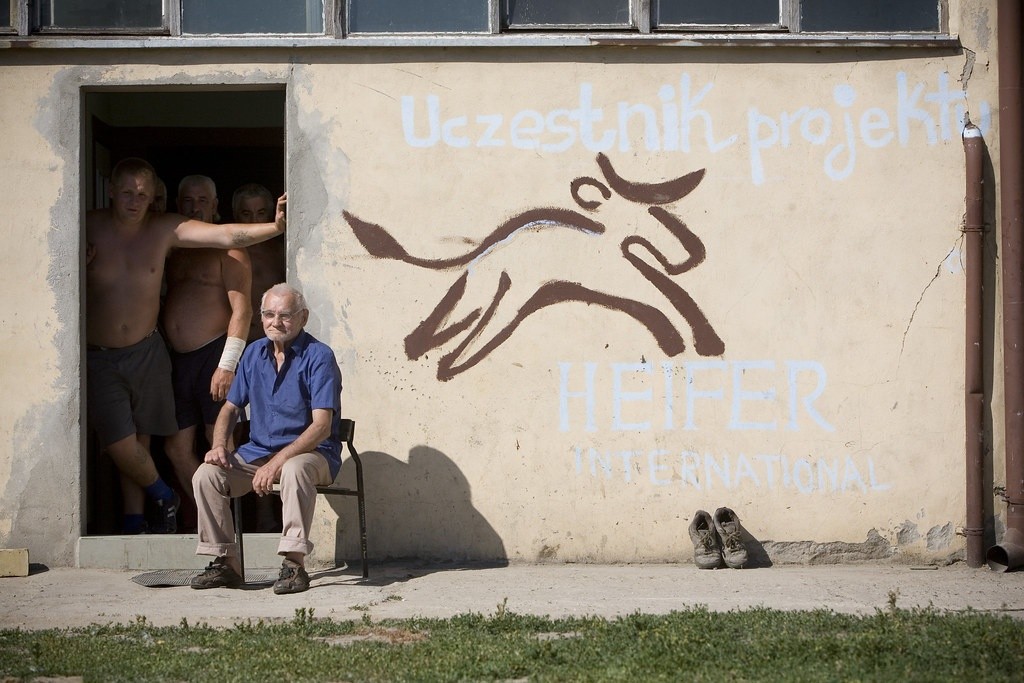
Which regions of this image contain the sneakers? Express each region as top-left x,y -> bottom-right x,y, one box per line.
713,507 -> 747,568
690,510 -> 722,570
274,558 -> 311,594
158,488 -> 180,534
191,560 -> 241,588
123,522 -> 149,535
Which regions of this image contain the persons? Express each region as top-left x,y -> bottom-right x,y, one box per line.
150,179 -> 167,212
164,174 -> 254,498
191,282 -> 342,595
86,157 -> 287,534
231,183 -> 286,345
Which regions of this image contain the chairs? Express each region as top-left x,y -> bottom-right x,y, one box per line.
231,420 -> 370,585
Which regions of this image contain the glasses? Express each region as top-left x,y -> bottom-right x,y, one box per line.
261,309 -> 304,323
